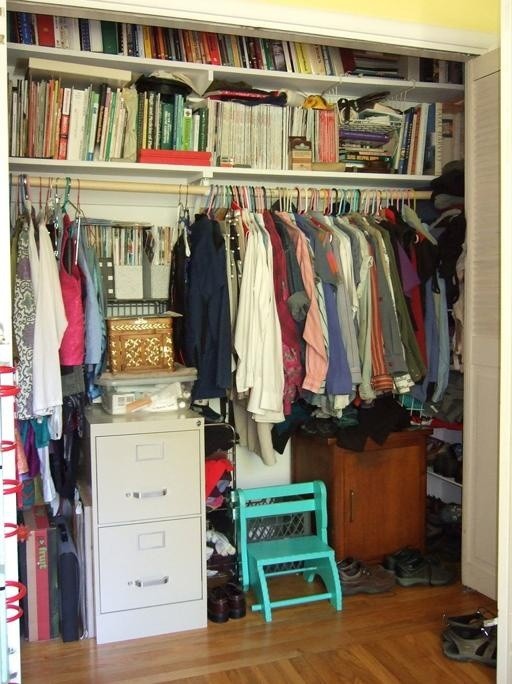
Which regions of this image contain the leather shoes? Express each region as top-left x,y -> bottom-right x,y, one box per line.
207,583 -> 245,623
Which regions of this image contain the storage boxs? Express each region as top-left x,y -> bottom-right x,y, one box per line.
426,465 -> 462,507
18,504 -> 60,642
432,425 -> 463,447
86,366 -> 199,418
102,311 -> 177,373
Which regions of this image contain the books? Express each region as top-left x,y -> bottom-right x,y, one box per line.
82,223 -> 176,266
6,9 -> 463,84
7,74 -> 463,176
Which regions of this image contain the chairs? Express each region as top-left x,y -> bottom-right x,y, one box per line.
229,476 -> 348,624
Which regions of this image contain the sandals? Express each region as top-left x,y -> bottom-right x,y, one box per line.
441,608 -> 497,667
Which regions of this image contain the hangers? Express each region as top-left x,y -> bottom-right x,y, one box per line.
170,179 -> 424,260
9,168 -> 100,264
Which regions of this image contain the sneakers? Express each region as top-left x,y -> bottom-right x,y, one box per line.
384,544 -> 456,587
425,494 -> 462,538
426,434 -> 463,484
337,559 -> 396,595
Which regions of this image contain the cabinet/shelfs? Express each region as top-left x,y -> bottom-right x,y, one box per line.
197,406 -> 246,606
80,399 -> 216,647
9,33 -> 469,190
285,422 -> 435,569
